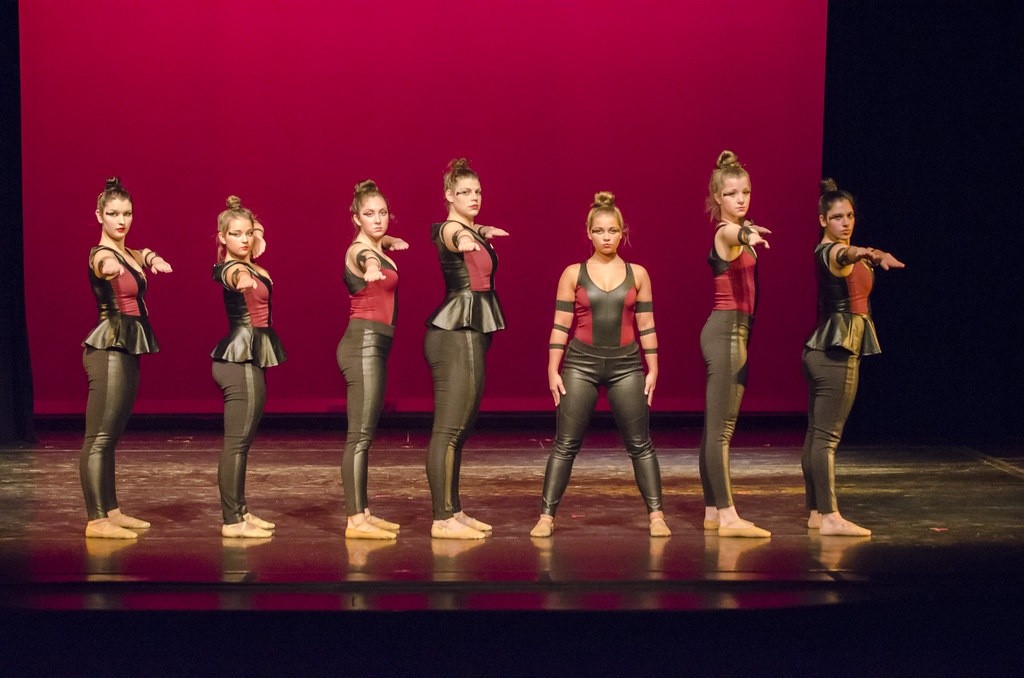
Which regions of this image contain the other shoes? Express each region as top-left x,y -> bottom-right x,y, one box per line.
718,518 -> 771,537
366,515 -> 400,530
243,512 -> 275,529
820,523 -> 872,536
431,522 -> 486,540
530,521 -> 554,536
704,519 -> 754,530
85,518 -> 138,539
107,508 -> 150,529
808,518 -> 820,529
650,519 -> 671,536
345,517 -> 397,539
222,522 -> 272,538
453,511 -> 492,531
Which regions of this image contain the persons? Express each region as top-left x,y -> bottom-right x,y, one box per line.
699,149 -> 773,538
208,195 -> 288,538
78,176 -> 174,540
527,191 -> 672,539
334,178 -> 410,540
800,179 -> 905,539
422,156 -> 510,540
